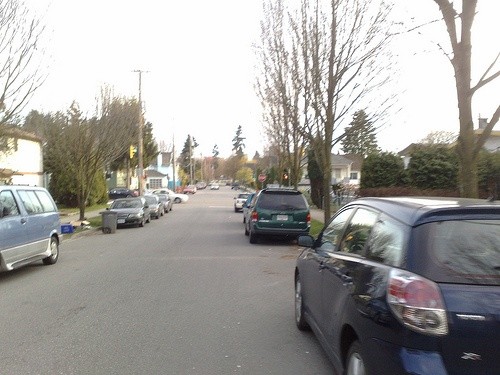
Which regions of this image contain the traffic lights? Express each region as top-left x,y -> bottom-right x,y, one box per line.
281,168 -> 288,180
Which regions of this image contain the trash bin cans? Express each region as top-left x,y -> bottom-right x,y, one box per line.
98,210 -> 118,234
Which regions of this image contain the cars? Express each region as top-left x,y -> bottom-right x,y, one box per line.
196,182 -> 206,190
106,197 -> 151,227
244,186 -> 311,244
210,183 -> 219,190
232,193 -> 251,212
108,187 -> 138,198
292,192 -> 500,375
157,193 -> 173,212
183,186 -> 194,193
143,189 -> 190,203
142,195 -> 164,219
188,185 -> 196,193
240,194 -> 256,223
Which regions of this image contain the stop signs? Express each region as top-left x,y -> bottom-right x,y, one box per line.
258,174 -> 266,182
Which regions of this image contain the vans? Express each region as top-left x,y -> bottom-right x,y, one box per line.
0,183 -> 62,273
